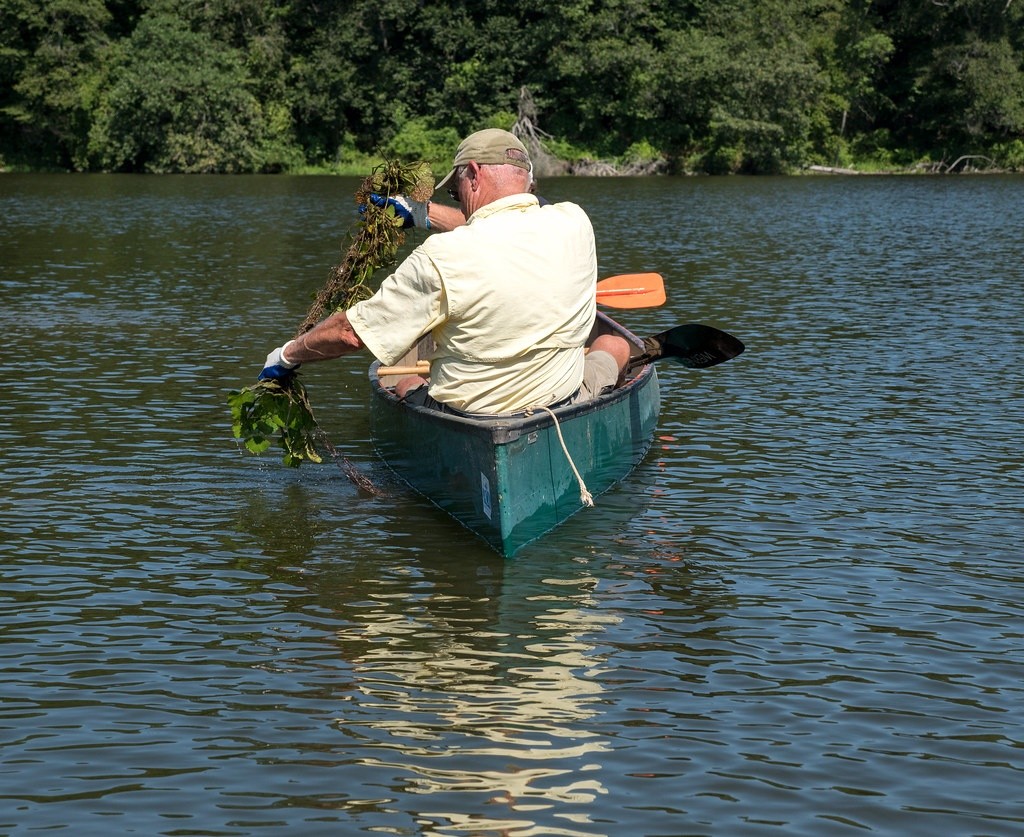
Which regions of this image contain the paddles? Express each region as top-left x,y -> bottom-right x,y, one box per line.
595,272 -> 669,311
625,322 -> 747,371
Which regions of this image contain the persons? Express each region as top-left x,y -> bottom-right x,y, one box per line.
257,127 -> 630,417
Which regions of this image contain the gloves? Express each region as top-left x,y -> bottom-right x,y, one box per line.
258,339 -> 302,382
358,195 -> 431,233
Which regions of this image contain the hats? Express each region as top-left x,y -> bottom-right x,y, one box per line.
435,128 -> 531,191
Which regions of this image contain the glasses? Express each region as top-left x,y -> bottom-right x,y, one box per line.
446,164 -> 482,203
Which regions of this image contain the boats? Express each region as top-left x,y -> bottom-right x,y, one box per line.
360,270 -> 662,564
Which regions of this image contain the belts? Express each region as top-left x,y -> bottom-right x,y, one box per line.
436,386 -> 582,418
